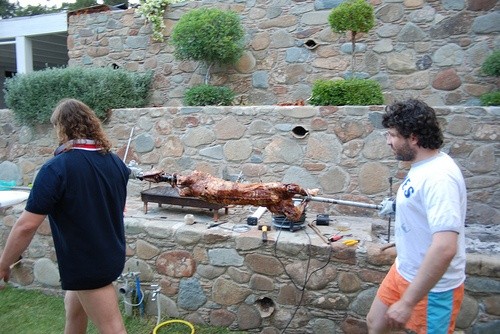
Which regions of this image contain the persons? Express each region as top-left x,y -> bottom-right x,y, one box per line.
0,98 -> 131,334
365,96 -> 466,334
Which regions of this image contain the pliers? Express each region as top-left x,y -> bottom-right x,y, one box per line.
343,239 -> 361,246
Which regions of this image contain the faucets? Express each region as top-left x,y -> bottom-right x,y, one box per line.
149,284 -> 162,301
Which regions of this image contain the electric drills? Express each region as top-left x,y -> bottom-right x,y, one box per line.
316,214 -> 333,227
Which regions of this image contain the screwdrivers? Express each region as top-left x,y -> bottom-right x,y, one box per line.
330,234 -> 352,242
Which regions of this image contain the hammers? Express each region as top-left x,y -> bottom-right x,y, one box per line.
258,224 -> 271,243
